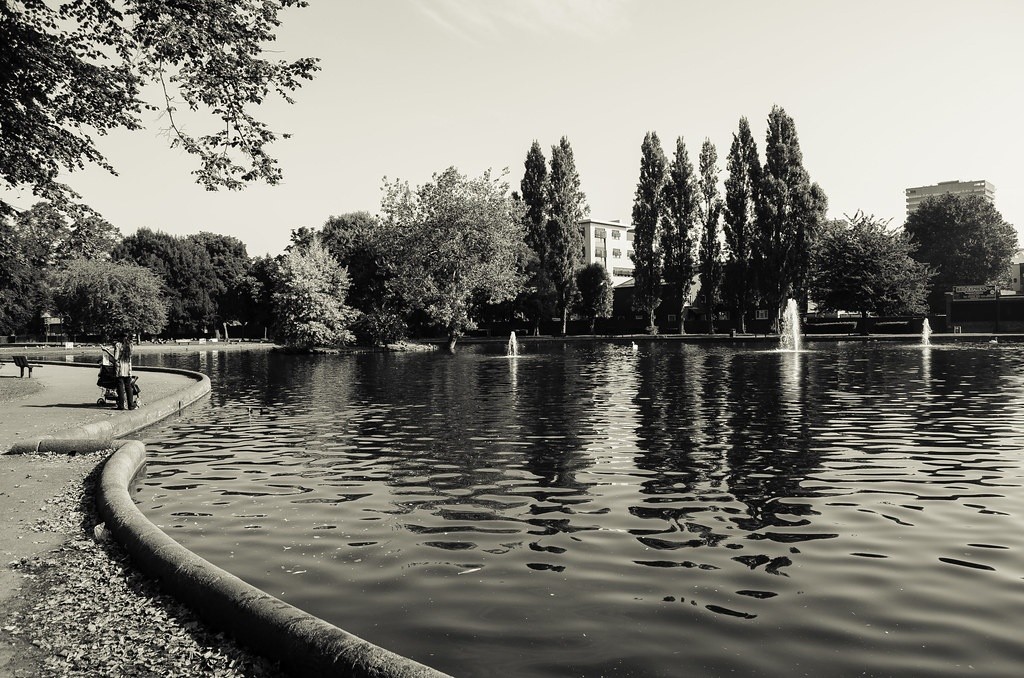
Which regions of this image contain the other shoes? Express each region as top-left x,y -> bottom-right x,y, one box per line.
134,406 -> 138,410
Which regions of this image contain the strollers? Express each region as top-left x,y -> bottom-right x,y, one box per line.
96,362 -> 142,408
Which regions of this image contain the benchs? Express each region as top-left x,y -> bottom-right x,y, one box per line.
12,356 -> 43,378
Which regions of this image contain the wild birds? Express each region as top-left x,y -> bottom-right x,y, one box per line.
632,341 -> 638,348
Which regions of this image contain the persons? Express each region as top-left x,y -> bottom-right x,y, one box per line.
109,329 -> 138,410
